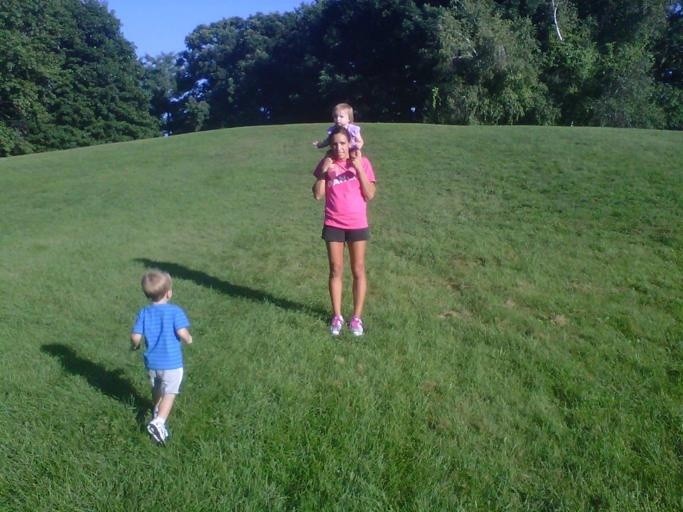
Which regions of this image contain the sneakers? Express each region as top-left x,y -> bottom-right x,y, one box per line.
329,314 -> 344,336
147,419 -> 166,448
349,317 -> 364,336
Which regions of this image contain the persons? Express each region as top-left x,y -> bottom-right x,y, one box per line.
129,270 -> 193,447
312,103 -> 364,181
312,127 -> 376,337
